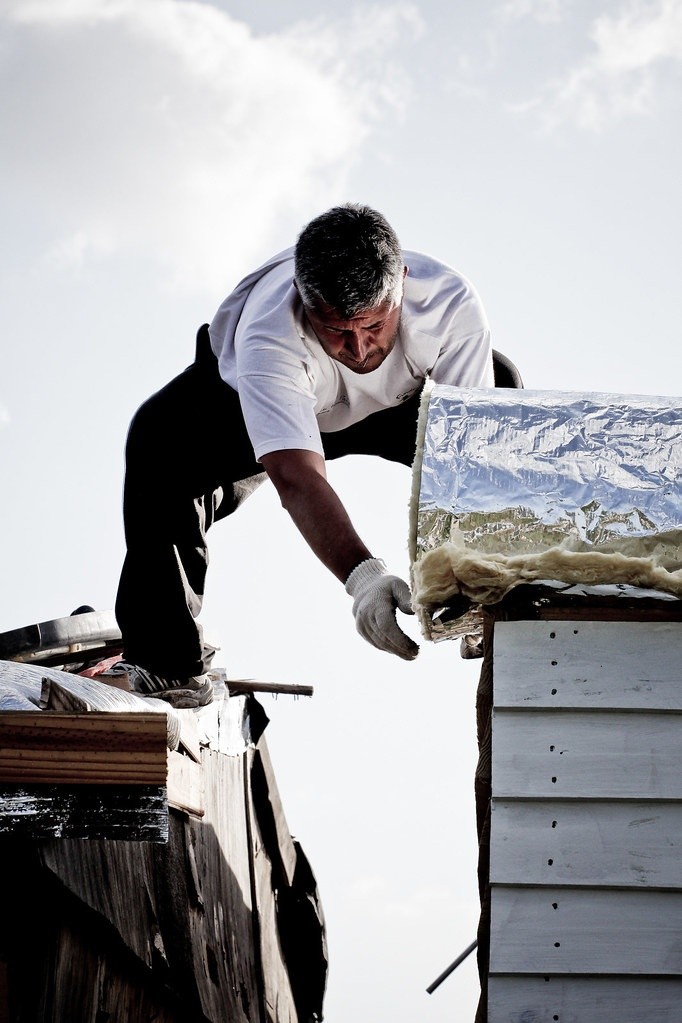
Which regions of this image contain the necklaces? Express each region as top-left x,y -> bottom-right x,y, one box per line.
358,358 -> 369,373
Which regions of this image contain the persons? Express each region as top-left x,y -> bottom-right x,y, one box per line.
93,205 -> 525,710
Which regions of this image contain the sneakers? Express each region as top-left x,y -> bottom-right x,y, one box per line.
99,660 -> 213,709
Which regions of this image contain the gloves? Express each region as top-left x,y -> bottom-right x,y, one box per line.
344,558 -> 423,662
